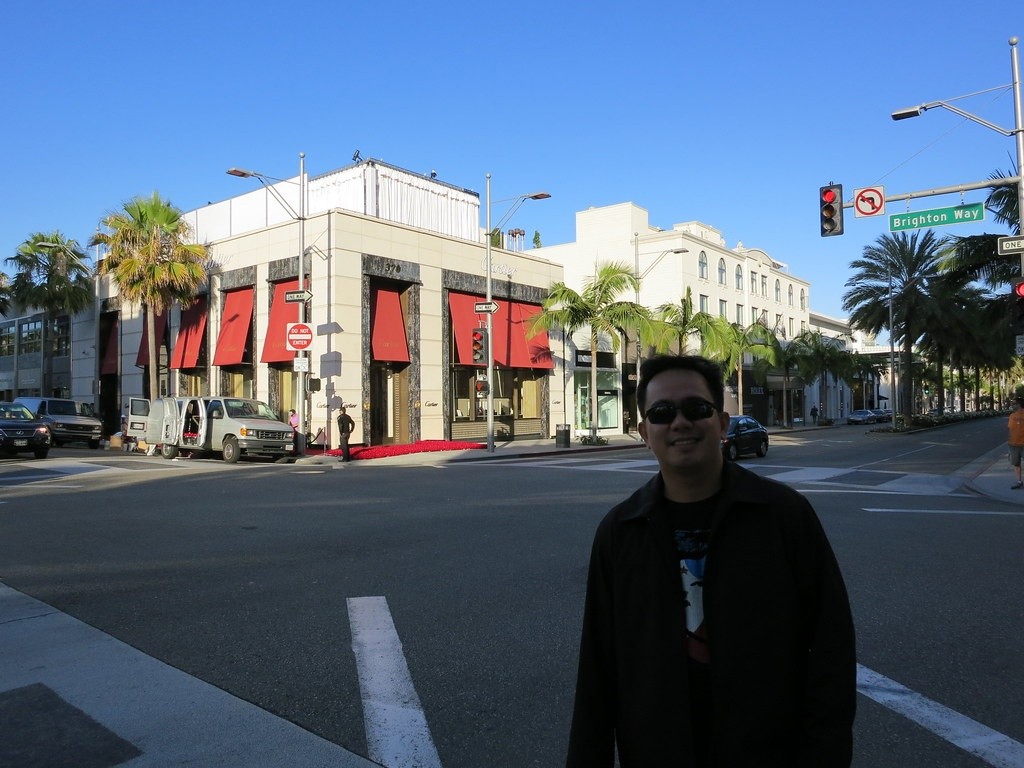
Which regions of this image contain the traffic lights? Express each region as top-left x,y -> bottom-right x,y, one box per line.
819,183 -> 844,238
471,328 -> 489,366
1010,276 -> 1024,300
925,385 -> 929,394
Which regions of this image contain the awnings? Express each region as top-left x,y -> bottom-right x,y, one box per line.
370,290 -> 409,361
169,296 -> 207,369
101,320 -> 118,373
260,278 -> 308,363
212,288 -> 253,366
449,292 -> 554,368
135,309 -> 167,365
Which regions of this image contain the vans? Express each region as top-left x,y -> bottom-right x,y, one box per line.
12,396 -> 103,450
126,394 -> 296,464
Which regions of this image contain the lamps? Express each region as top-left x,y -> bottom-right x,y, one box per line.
352,150 -> 363,162
431,170 -> 437,179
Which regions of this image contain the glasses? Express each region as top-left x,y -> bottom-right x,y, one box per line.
643,400 -> 720,423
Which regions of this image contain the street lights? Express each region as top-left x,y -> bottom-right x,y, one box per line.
634,231 -> 690,442
34,225 -> 101,418
485,172 -> 553,455
224,151 -> 307,459
891,36 -> 1024,304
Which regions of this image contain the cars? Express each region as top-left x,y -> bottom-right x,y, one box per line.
0,401 -> 53,459
847,405 -> 971,426
721,415 -> 770,461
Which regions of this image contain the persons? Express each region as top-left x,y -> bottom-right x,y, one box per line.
289,409 -> 299,451
564,354 -> 857,768
810,405 -> 818,424
337,407 -> 355,462
1007,398 -> 1024,488
6,411 -> 13,418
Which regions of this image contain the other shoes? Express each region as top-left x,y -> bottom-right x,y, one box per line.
1010,481 -> 1023,490
338,459 -> 349,462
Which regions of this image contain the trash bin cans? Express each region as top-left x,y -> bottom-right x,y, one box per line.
557,422 -> 571,449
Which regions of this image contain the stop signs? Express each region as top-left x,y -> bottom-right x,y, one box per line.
286,322 -> 318,351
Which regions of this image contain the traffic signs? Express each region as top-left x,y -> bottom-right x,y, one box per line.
285,290 -> 313,302
473,301 -> 499,314
